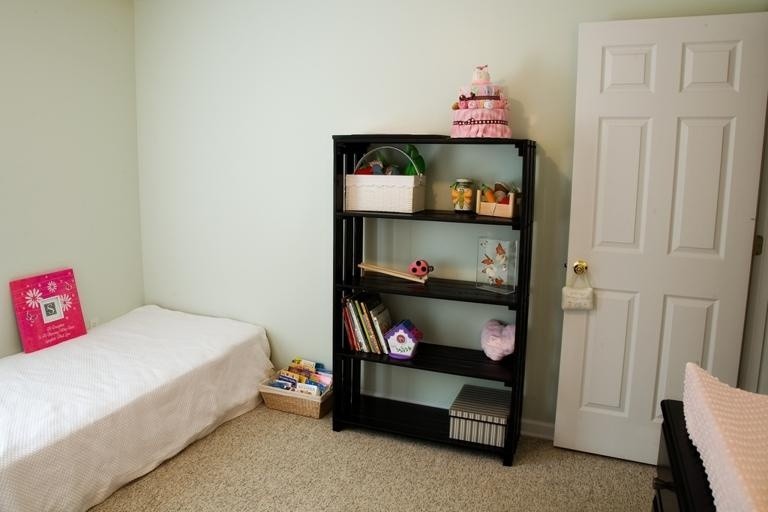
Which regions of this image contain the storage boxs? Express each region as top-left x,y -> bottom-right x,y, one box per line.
448,385 -> 512,447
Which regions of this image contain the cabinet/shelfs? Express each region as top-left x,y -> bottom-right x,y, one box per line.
330,134 -> 537,466
649,398 -> 716,512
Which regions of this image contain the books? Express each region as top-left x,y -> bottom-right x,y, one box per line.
343,296 -> 392,354
270,356 -> 334,396
10,268 -> 86,355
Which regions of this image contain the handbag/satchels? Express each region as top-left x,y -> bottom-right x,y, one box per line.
561,273 -> 594,310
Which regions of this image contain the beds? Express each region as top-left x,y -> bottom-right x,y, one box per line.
0,305 -> 275,512
